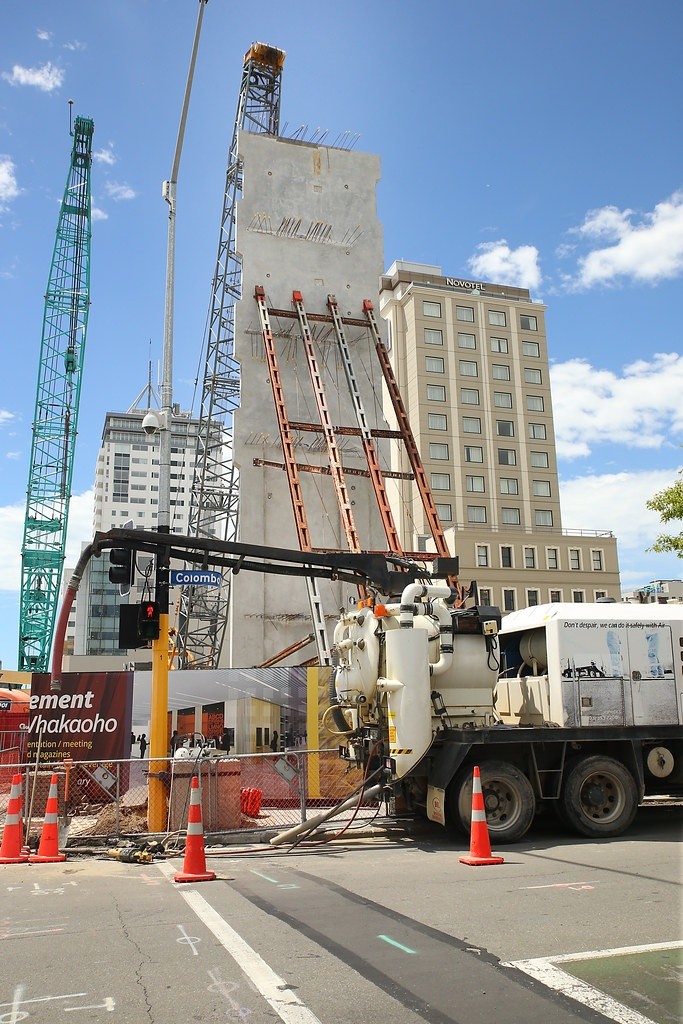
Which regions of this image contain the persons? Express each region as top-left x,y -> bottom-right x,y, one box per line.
137,735 -> 141,741
270,731 -> 278,760
646,628 -> 663,678
170,730 -> 183,757
131,732 -> 135,744
212,728 -> 231,755
606,630 -> 624,677
140,734 -> 149,758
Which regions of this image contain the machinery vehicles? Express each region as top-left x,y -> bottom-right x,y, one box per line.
49,527 -> 683,845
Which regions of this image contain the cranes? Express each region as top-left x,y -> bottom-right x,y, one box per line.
17,99 -> 94,672
170,40 -> 287,673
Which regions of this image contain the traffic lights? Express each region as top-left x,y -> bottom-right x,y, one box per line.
108,519 -> 135,596
139,599 -> 160,640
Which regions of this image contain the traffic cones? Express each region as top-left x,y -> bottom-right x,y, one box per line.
0,773 -> 30,864
458,766 -> 504,866
28,774 -> 66,863
173,776 -> 217,881
